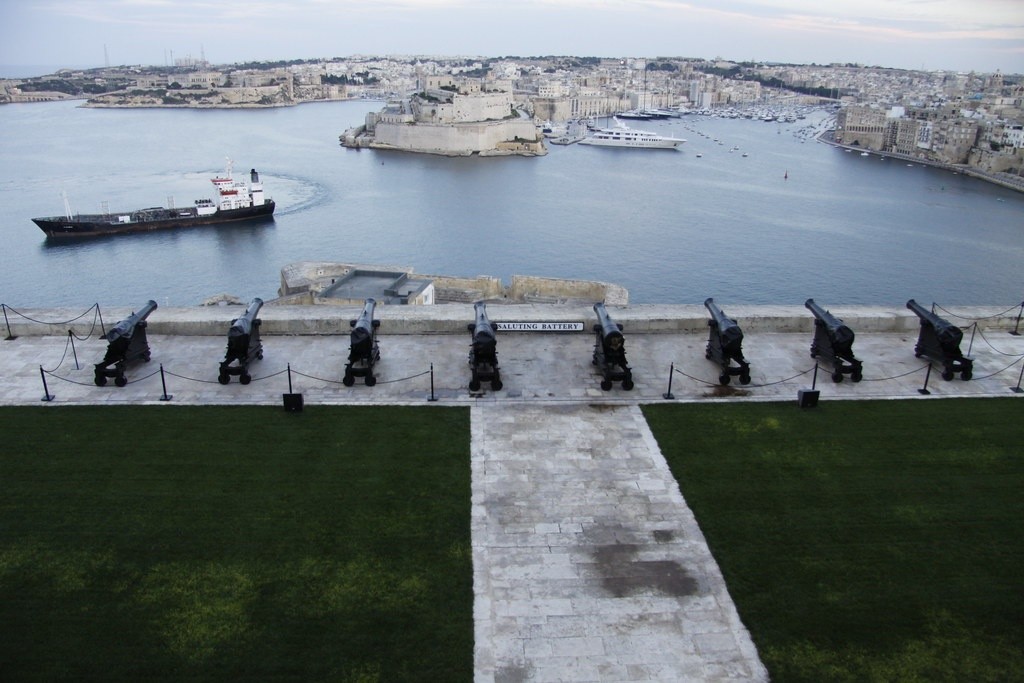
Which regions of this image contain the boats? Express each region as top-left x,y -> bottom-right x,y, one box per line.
29,156 -> 275,240
534,104 -> 827,150
